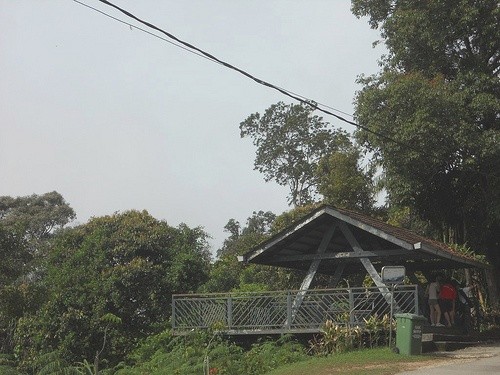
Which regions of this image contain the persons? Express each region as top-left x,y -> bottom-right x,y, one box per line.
424,277 -> 444,326
438,278 -> 457,327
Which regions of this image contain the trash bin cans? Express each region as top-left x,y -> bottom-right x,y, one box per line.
393,312 -> 425,355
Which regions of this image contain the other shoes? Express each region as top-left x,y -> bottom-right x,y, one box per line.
436,323 -> 445,326
431,324 -> 435,326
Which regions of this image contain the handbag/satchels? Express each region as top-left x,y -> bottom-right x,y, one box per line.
425,289 -> 429,297
